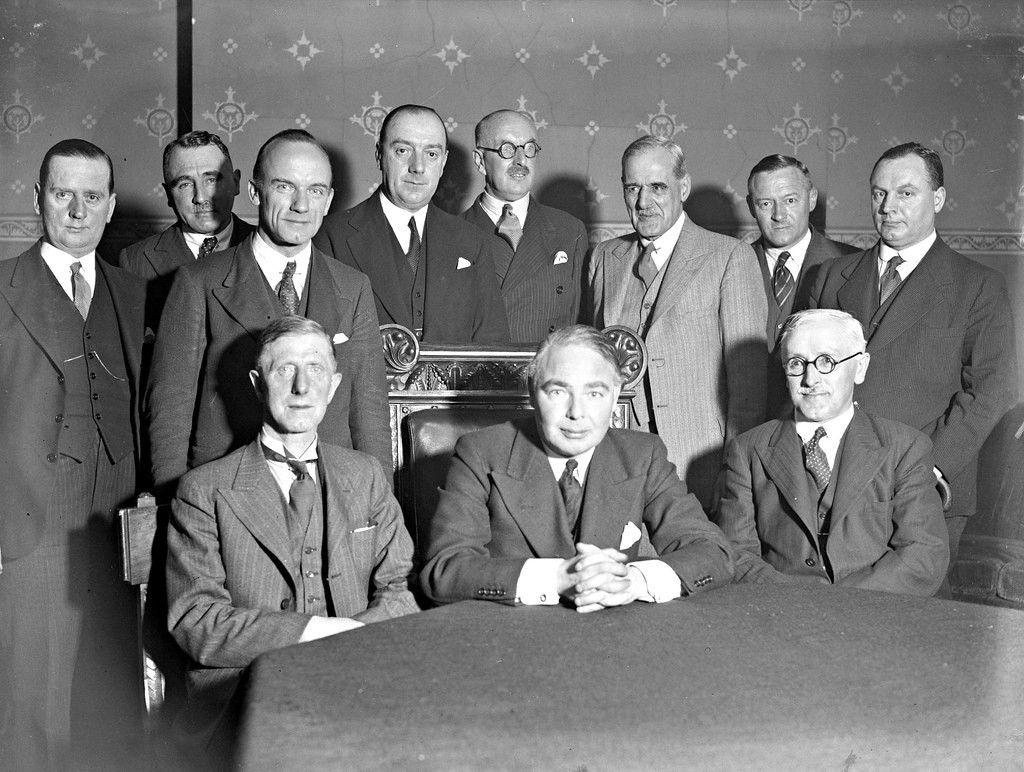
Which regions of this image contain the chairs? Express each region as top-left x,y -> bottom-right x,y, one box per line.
119,491 -> 187,772
381,322 -> 647,606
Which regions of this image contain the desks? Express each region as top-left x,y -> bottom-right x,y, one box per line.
248,586 -> 1024,772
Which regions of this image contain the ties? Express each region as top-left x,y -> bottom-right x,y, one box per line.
771,251 -> 795,312
259,440 -> 316,533
803,426 -> 832,495
499,204 -> 523,252
70,262 -> 92,322
274,261 -> 300,315
557,458 -> 582,533
637,243 -> 658,290
405,217 -> 421,279
198,239 -> 216,262
880,256 -> 906,307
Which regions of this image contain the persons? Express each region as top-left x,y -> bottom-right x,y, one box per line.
119,130 -> 259,330
311,105 -> 510,343
420,324 -> 735,612
0,138 -> 171,772
710,309 -> 951,597
589,135 -> 773,526
142,128 -> 394,702
166,312 -> 424,772
457,109 -> 589,343
746,154 -> 865,346
807,142 -> 1018,600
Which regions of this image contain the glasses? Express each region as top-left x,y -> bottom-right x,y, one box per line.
477,141 -> 541,159
779,352 -> 862,376
